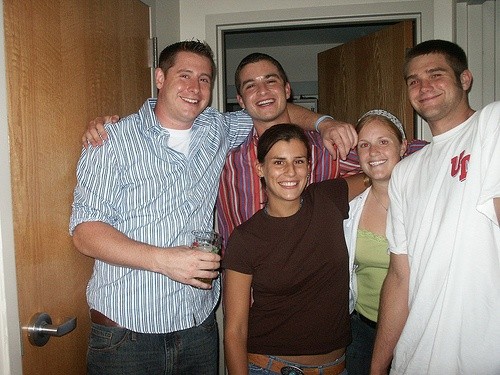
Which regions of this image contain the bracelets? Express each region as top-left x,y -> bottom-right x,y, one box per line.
314,114 -> 335,134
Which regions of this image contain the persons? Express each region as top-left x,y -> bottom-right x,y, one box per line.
367,40 -> 500,375
69,39 -> 359,375
222,124 -> 374,374
342,110 -> 409,375
82,52 -> 431,259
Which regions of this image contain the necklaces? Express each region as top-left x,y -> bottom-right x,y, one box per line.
371,187 -> 393,212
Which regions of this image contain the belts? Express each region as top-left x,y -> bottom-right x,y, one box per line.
245,351 -> 347,375
90,308 -> 120,328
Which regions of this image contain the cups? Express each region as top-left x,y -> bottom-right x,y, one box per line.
190,230 -> 221,286
